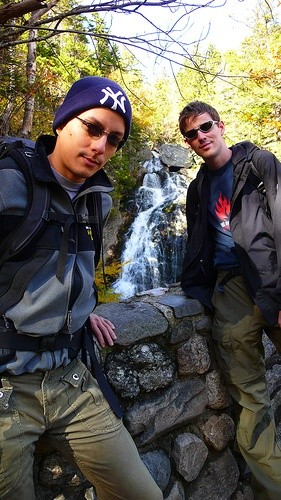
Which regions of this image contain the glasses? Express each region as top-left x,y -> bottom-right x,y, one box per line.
73,115 -> 123,148
185,121 -> 218,140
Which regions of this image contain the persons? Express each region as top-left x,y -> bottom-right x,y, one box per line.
0,77 -> 163,500
179,101 -> 281,500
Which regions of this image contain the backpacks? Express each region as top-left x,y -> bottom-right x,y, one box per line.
1,135 -> 126,419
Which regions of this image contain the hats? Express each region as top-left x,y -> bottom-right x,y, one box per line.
52,76 -> 131,152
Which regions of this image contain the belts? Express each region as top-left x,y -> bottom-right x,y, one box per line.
216,267 -> 244,292
